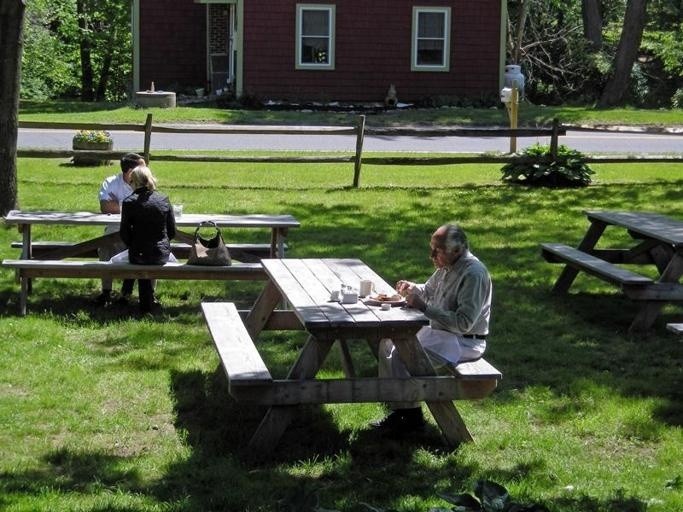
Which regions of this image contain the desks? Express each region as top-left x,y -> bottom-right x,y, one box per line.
552,213 -> 683,339
5,210 -> 300,316
215,259 -> 474,463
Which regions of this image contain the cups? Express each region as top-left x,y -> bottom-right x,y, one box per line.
360,278 -> 375,298
173,202 -> 183,218
343,293 -> 358,304
332,290 -> 340,299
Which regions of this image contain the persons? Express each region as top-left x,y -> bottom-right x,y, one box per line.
95,153 -> 157,306
370,223 -> 492,433
121,166 -> 176,308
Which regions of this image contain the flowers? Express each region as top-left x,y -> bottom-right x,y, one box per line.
74,128 -> 112,143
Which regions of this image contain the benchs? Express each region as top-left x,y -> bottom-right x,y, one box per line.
2,260 -> 267,278
540,243 -> 657,299
438,358 -> 502,400
200,301 -> 286,407
666,323 -> 683,336
9,240 -> 288,259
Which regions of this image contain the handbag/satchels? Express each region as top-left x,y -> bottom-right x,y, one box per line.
185,219 -> 233,267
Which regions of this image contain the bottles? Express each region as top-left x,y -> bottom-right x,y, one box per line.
340,284 -> 353,293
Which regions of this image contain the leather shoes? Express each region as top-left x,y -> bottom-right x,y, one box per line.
367,406 -> 426,431
96,291 -> 163,311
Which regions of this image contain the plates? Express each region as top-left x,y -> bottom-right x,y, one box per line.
365,294 -> 405,304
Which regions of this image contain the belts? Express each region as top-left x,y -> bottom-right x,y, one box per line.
458,332 -> 490,342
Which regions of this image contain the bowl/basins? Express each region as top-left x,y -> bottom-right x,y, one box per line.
381,304 -> 393,309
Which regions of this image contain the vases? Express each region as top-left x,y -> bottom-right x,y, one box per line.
73,139 -> 113,168
